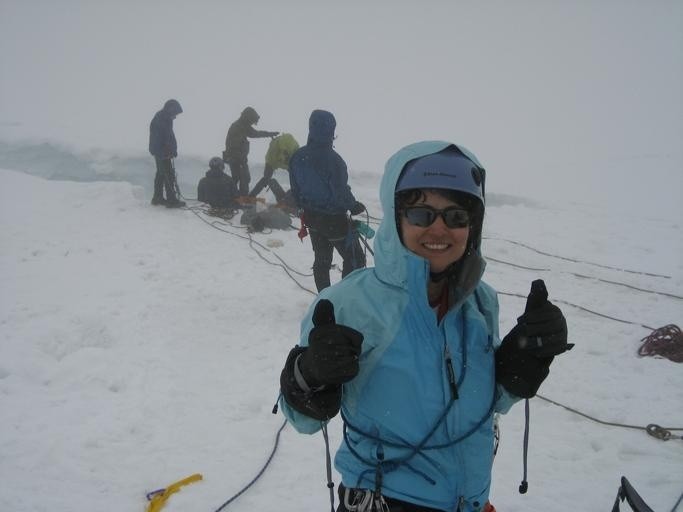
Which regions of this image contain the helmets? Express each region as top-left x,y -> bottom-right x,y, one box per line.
386,139 -> 485,211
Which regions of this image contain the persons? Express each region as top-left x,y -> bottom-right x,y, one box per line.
196,156 -> 254,211
147,98 -> 186,208
287,108 -> 366,295
275,138 -> 568,512
249,133 -> 302,199
220,104 -> 281,198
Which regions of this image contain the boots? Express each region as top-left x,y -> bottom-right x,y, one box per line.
151,188 -> 167,205
166,192 -> 186,208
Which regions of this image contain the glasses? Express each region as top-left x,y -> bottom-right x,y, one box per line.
394,206 -> 475,228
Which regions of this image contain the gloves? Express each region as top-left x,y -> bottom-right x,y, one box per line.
350,201 -> 366,215
495,279 -> 575,399
280,298 -> 365,422
